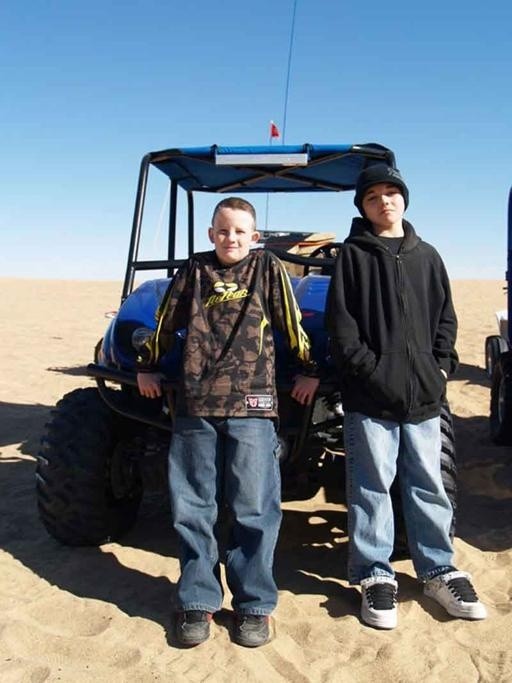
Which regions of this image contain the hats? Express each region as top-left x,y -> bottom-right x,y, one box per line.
353,163 -> 409,217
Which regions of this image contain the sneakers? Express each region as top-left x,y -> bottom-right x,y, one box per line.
233,613 -> 273,647
175,610 -> 213,645
360,577 -> 400,630
423,571 -> 487,619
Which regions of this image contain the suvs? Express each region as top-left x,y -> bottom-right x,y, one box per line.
36,146 -> 460,549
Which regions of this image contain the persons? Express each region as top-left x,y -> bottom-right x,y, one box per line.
323,162 -> 492,629
136,194 -> 322,648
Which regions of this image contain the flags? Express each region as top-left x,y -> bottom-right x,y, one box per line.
270,122 -> 281,138
